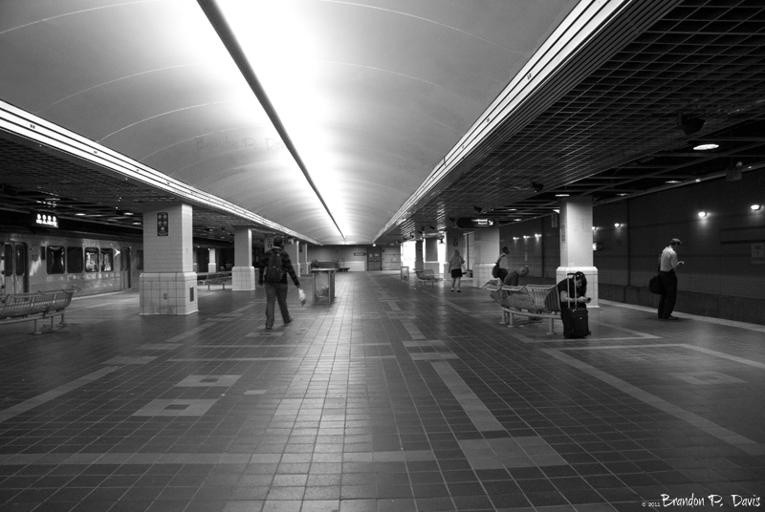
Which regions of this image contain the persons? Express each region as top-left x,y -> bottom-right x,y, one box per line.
256,237 -> 301,333
544,271 -> 592,336
447,248 -> 466,293
655,237 -> 685,321
502,264 -> 542,321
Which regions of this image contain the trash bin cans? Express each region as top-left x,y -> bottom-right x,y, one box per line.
400,266 -> 409,281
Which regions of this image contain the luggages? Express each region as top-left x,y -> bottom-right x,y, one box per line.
562,271 -> 590,340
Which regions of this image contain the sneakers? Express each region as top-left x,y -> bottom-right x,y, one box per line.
450,288 -> 462,293
265,317 -> 293,330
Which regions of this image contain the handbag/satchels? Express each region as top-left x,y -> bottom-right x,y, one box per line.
647,273 -> 664,295
297,287 -> 307,306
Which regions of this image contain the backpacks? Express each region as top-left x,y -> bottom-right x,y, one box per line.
264,248 -> 287,284
492,255 -> 505,278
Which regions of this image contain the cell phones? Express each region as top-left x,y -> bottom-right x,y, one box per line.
587,297 -> 591,301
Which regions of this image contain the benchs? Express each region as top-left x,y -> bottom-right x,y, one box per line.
414,267 -> 443,289
481,284 -> 564,335
1,288 -> 80,337
